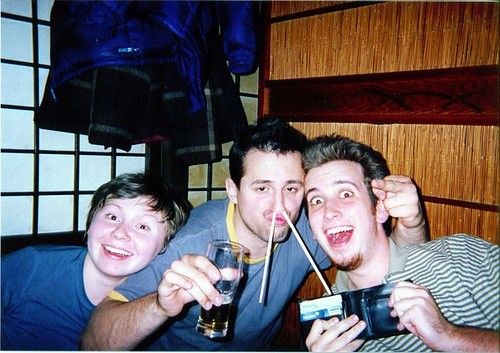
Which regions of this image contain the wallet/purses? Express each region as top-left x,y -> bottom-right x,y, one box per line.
299,279 -> 416,341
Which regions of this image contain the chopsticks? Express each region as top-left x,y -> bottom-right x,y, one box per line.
258,213 -> 331,303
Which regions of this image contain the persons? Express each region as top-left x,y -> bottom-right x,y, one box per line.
79,119 -> 429,351
304,138 -> 500,353
0,172 -> 190,351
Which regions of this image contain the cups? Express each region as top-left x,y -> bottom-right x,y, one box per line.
195,239 -> 244,339
